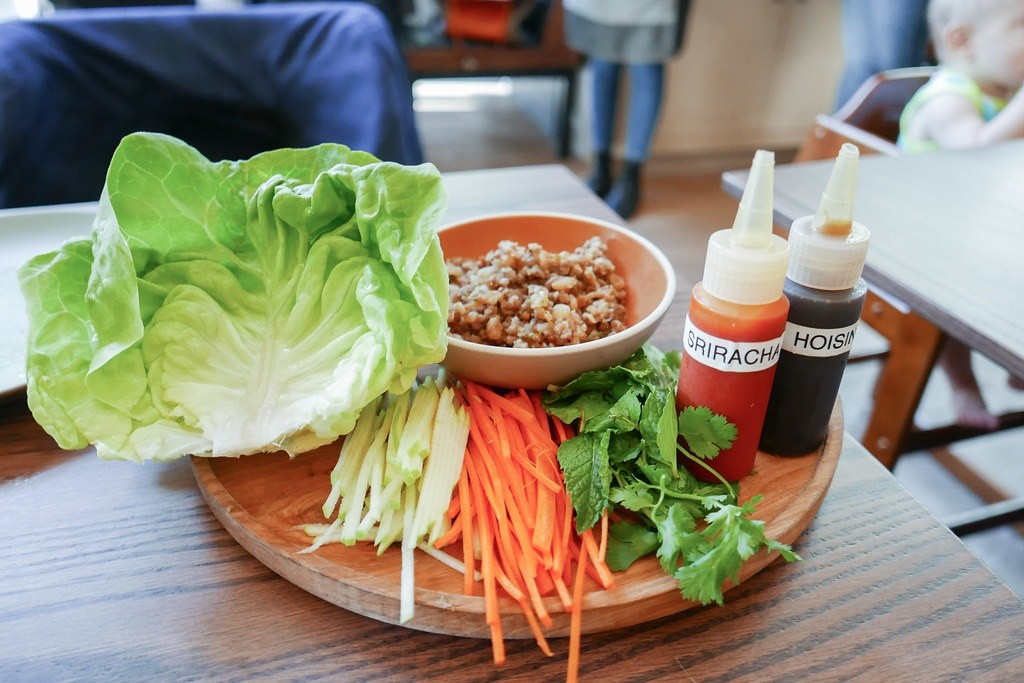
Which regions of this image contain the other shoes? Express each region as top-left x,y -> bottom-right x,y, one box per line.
588,153 -> 639,218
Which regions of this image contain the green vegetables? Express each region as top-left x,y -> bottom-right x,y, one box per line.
539,343 -> 804,605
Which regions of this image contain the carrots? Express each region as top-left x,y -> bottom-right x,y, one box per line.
420,376 -> 624,683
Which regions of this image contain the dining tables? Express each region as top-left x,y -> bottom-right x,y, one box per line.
1,165 -> 1024,683
719,137 -> 1023,536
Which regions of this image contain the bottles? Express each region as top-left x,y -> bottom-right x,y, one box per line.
675,152 -> 790,484
758,144 -> 872,458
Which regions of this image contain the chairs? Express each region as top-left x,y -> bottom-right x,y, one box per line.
789,65 -> 949,472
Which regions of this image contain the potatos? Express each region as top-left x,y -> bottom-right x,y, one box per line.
299,367 -> 472,624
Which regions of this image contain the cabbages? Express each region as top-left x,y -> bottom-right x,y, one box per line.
19,132 -> 452,461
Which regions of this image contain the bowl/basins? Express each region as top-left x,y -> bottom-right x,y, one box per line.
436,214 -> 675,386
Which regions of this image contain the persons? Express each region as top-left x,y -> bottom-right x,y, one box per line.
831,0 -> 929,114
561,0 -> 690,220
895,0 -> 1024,428
